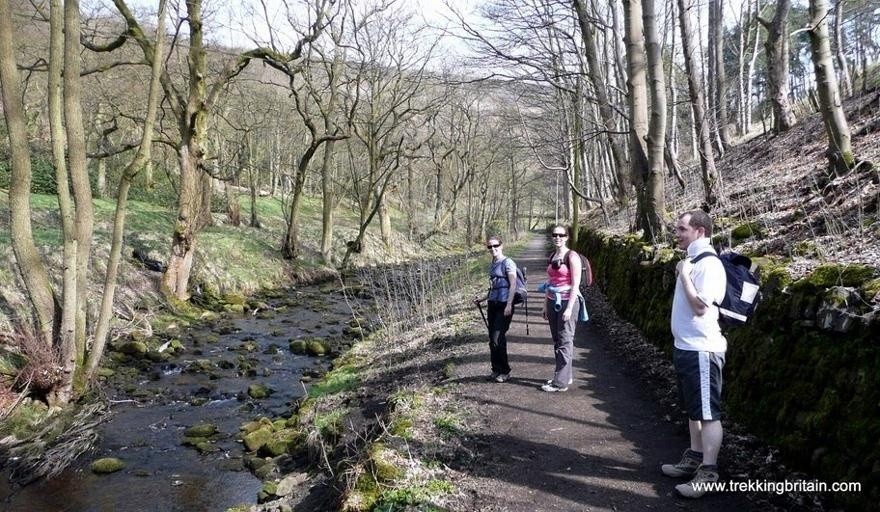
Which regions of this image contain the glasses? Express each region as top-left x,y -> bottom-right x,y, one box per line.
552,232 -> 566,238
487,245 -> 499,249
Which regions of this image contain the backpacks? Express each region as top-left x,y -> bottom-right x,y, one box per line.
691,252 -> 760,329
549,249 -> 593,288
490,258 -> 526,305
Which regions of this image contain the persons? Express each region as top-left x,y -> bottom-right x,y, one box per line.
541,225 -> 583,392
474,236 -> 516,382
662,210 -> 729,500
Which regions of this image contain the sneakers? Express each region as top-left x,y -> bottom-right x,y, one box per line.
486,372 -> 510,382
675,464 -> 719,498
542,377 -> 573,392
661,450 -> 704,477
